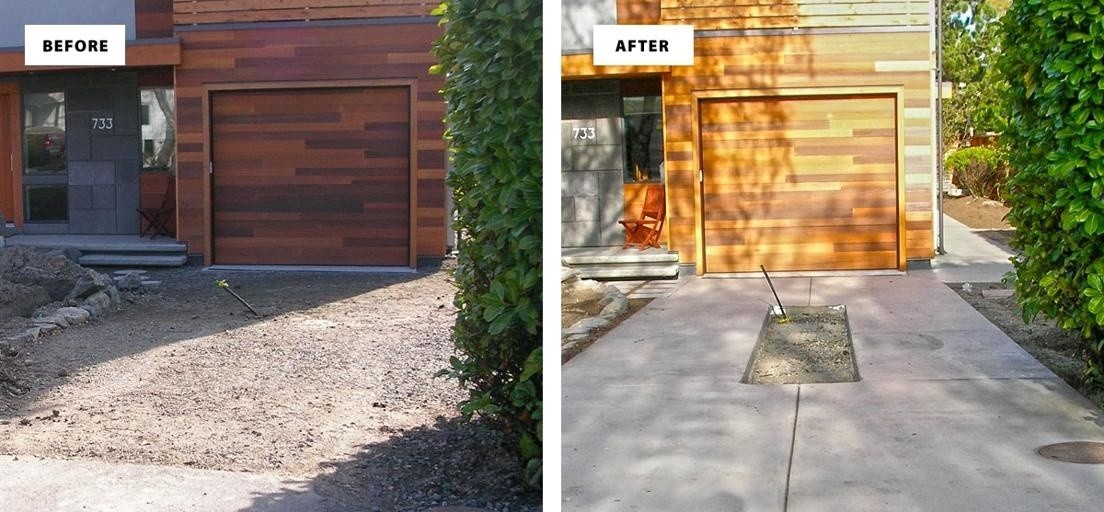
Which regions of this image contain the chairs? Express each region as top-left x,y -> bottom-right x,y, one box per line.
618,184 -> 666,250
136,175 -> 175,239
